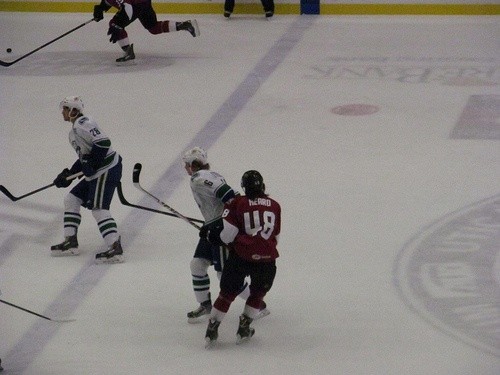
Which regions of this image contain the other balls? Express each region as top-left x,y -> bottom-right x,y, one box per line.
7,49 -> 11,52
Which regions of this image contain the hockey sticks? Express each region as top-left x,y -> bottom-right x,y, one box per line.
0,300 -> 75,322
0,171 -> 83,201
117,181 -> 204,223
132,163 -> 200,230
0,18 -> 95,67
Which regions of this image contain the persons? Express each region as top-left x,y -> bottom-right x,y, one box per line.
92,0 -> 201,66
182,147 -> 269,324
205,169 -> 281,347
223,0 -> 275,21
50,96 -> 126,264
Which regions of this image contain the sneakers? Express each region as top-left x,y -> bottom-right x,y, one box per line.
51,235 -> 79,256
236,313 -> 256,344
115,43 -> 137,66
95,241 -> 124,263
187,300 -> 213,322
177,18 -> 200,40
223,10 -> 231,21
203,319 -> 221,347
264,10 -> 274,20
255,299 -> 270,319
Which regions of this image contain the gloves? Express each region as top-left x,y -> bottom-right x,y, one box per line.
107,22 -> 123,43
94,5 -> 104,22
83,160 -> 98,177
54,168 -> 72,188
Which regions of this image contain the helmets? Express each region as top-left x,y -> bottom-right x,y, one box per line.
63,95 -> 85,113
183,146 -> 208,164
241,170 -> 265,198
